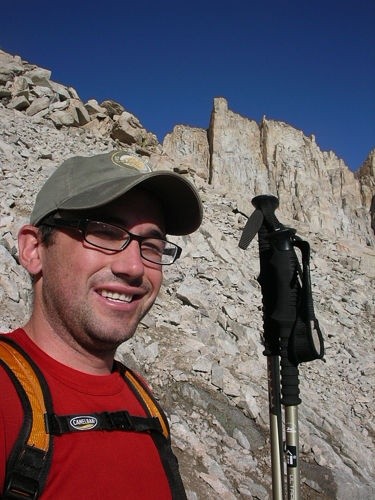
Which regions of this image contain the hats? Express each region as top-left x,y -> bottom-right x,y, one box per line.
30,149 -> 204,235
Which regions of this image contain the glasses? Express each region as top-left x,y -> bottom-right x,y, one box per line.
40,214 -> 183,266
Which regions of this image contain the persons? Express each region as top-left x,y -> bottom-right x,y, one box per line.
0,149 -> 204,500
